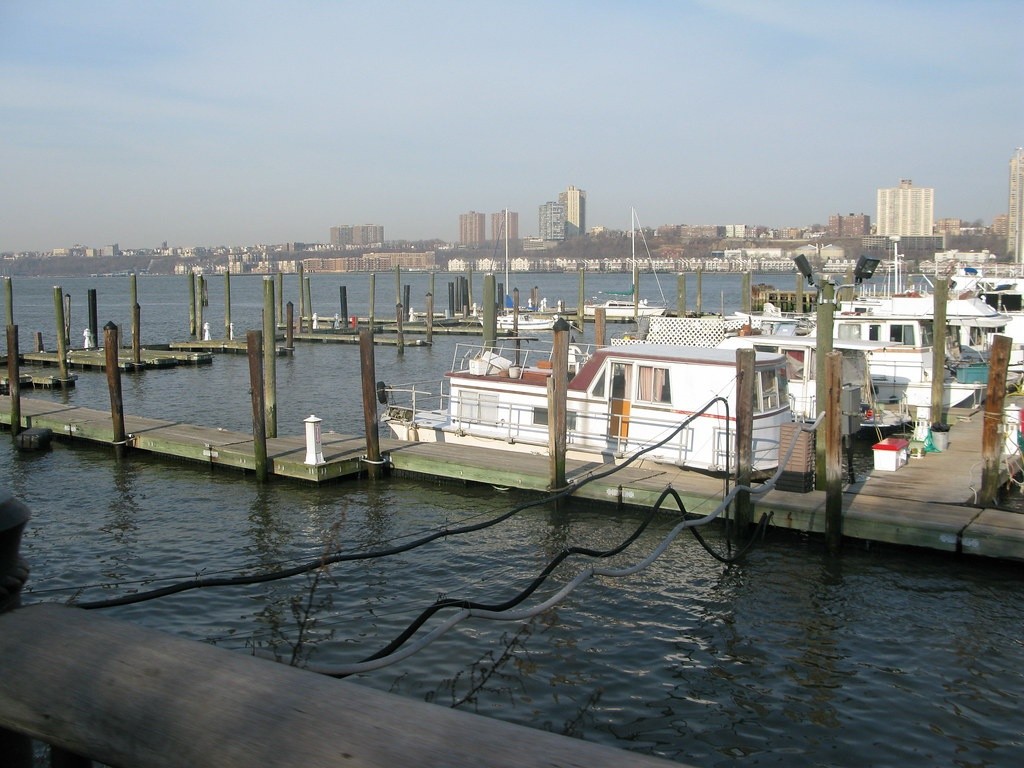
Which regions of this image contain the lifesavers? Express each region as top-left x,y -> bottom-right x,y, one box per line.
377,380 -> 389,405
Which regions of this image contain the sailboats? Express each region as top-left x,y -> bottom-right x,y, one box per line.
583,207 -> 669,321
474,209 -> 577,332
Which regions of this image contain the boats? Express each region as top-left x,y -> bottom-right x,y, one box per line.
608,235 -> 1024,432
378,326 -> 807,480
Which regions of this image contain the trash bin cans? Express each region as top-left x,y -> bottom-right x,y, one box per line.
931,425 -> 950,451
